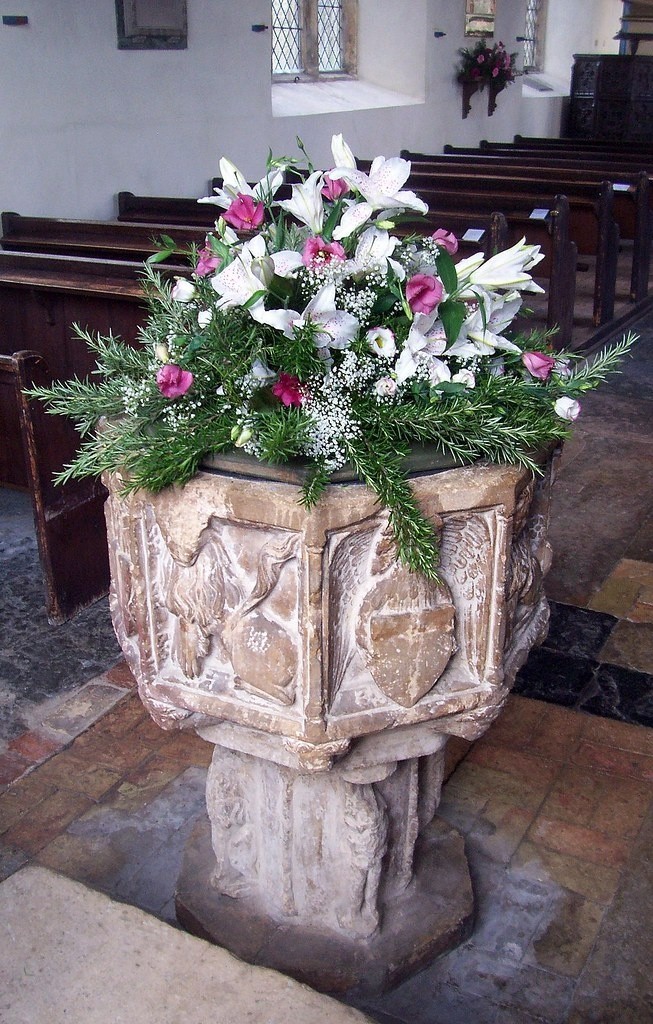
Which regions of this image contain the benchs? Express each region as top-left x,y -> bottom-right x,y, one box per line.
0,135 -> 653,626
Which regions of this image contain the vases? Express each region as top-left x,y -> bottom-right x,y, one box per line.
96,410 -> 565,997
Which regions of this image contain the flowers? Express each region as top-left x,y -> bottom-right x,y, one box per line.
21,134 -> 640,585
458,37 -> 519,92
